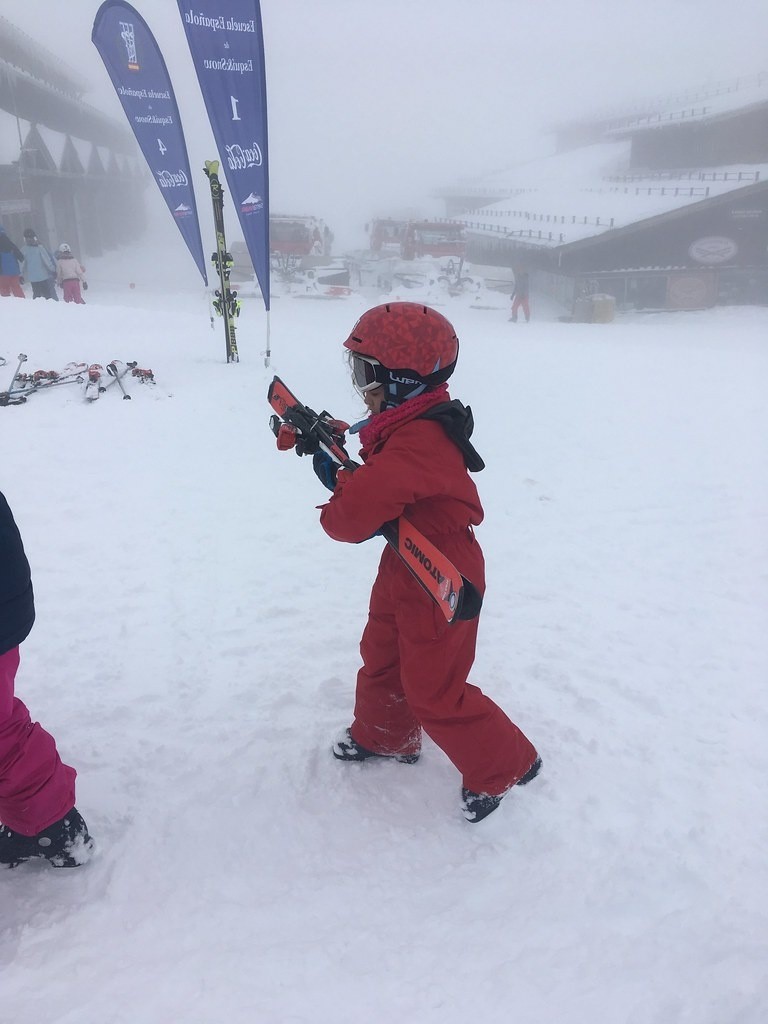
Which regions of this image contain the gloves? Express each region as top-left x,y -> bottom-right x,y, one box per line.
83,282 -> 88,291
19,277 -> 25,285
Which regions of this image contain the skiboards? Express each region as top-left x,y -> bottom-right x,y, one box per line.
203,158 -> 241,363
8,361 -> 89,399
264,373 -> 483,626
84,359 -> 174,403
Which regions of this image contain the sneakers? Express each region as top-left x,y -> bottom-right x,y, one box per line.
459,754 -> 544,822
0,807 -> 91,869
331,729 -> 420,765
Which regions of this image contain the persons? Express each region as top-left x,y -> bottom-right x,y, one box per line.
0,492 -> 95,869
509,265 -> 529,322
315,302 -> 542,824
0,227 -> 87,304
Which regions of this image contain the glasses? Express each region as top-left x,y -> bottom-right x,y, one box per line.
350,355 -> 384,392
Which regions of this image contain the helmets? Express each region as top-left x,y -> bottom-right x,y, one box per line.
60,244 -> 72,253
343,301 -> 458,404
24,229 -> 36,238
